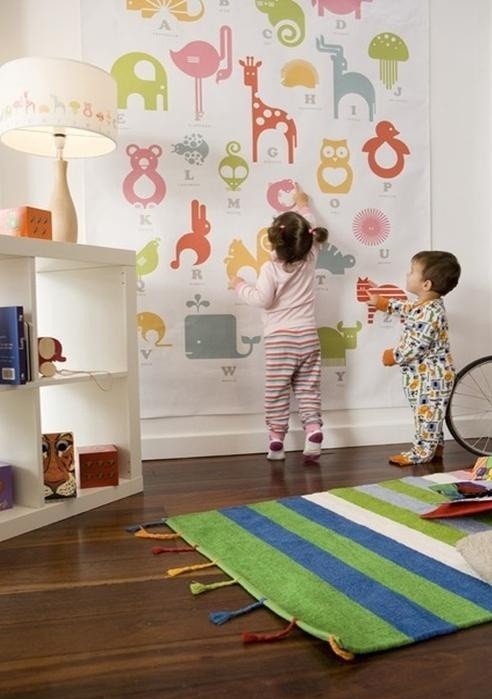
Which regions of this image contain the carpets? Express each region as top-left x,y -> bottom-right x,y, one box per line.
127,464 -> 491,665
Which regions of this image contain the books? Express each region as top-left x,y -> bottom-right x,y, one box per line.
1,306 -> 32,386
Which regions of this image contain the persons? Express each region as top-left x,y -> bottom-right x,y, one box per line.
228,181 -> 328,462
365,250 -> 461,467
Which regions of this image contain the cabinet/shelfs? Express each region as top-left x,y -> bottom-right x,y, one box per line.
0,233 -> 144,543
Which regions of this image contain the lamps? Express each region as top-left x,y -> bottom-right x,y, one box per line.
0,54 -> 117,245
